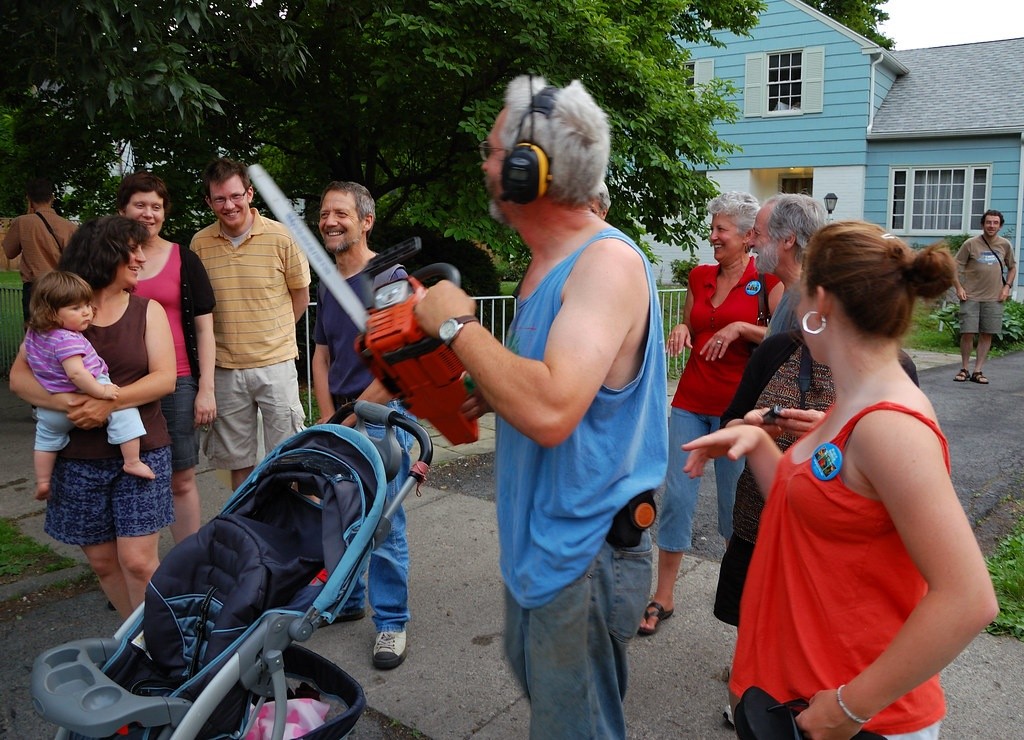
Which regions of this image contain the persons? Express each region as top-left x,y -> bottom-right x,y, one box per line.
7,225 -> 177,621
188,156 -> 313,493
413,76 -> 666,740
308,181 -> 420,668
952,209 -> 1016,384
683,219 -> 1001,740
24,269 -> 156,501
107,173 -> 217,612
638,192 -> 918,729
3,176 -> 78,421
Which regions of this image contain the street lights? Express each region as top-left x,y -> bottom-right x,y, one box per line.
824,193 -> 838,225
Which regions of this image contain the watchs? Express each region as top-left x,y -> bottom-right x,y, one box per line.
436,315 -> 478,348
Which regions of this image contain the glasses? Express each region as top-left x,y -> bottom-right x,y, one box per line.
479,141 -> 513,161
211,184 -> 251,206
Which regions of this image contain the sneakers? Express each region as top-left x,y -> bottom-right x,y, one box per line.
317,606 -> 366,628
373,630 -> 407,669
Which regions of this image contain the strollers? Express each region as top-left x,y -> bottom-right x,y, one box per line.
28,400 -> 434,740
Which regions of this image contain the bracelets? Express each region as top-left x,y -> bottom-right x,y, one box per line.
1006,282 -> 1013,288
835,685 -> 872,724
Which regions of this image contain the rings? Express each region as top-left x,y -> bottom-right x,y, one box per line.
669,339 -> 674,342
716,340 -> 722,344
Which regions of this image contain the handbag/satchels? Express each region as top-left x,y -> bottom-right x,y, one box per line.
733,686 -> 888,740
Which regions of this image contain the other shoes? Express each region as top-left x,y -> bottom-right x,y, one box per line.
723,705 -> 736,729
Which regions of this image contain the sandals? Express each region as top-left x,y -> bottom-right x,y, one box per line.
970,372 -> 989,384
953,368 -> 970,381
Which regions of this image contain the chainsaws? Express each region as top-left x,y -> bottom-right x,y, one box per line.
249,163 -> 479,447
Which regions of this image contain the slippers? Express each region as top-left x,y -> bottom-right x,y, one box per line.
637,602 -> 673,637
